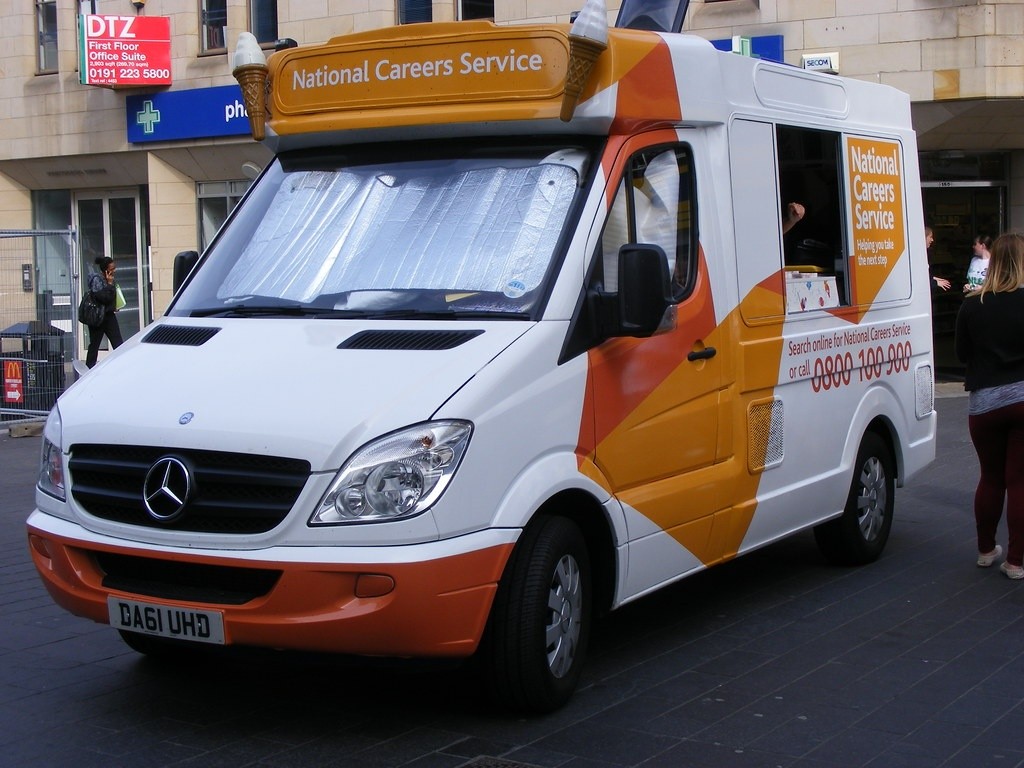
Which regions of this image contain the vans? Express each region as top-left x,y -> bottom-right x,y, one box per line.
25,0 -> 938,724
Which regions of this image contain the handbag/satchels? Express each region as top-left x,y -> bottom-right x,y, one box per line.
77,275 -> 105,327
114,283 -> 127,311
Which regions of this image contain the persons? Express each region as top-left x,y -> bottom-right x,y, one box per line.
963,235 -> 993,293
85,256 -> 123,368
955,233 -> 1024,580
782,202 -> 805,234
925,226 -> 951,291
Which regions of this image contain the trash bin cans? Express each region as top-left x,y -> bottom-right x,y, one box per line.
0,320 -> 66,421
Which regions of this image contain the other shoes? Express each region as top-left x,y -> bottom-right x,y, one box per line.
999,562 -> 1024,580
977,544 -> 1002,566
